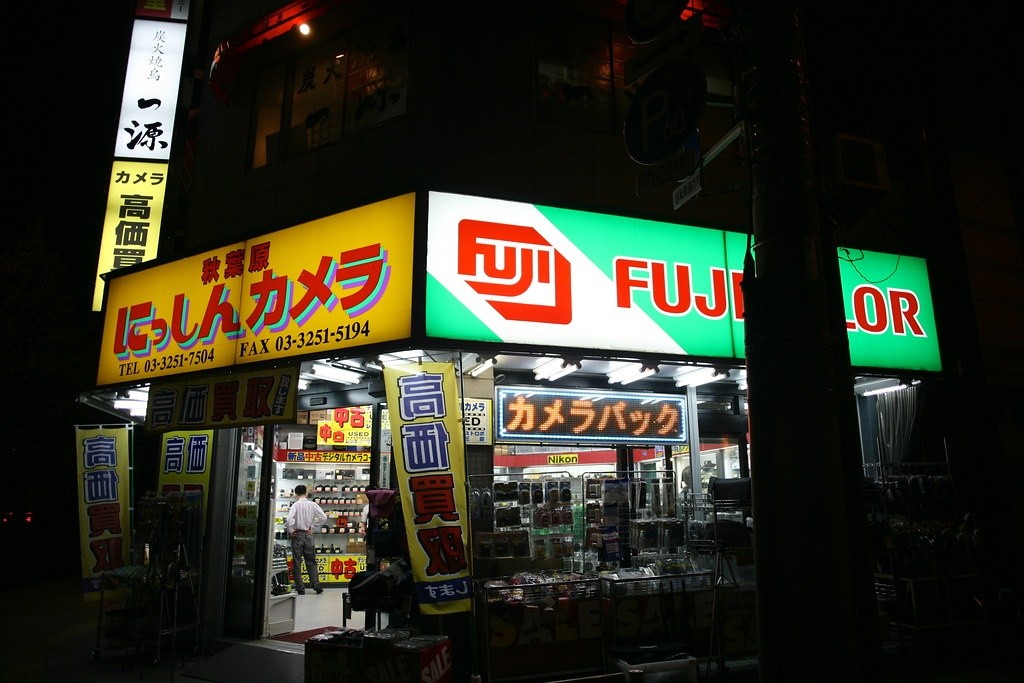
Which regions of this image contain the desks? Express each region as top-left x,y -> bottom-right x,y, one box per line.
304,627 -> 452,683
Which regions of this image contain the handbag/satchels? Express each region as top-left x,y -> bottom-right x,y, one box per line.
708,477 -> 751,508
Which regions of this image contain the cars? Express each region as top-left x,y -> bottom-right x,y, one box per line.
0,505 -> 37,532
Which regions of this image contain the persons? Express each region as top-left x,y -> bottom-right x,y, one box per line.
358,485 -> 378,538
286,484 -> 327,594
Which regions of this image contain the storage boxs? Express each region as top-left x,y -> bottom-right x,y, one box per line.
707,476 -> 752,503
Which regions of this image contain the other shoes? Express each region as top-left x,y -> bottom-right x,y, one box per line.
316,589 -> 323,594
298,590 -> 305,595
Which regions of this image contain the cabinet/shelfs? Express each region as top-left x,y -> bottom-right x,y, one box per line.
91,489 -> 205,669
581,470 -> 724,683
863,436 -> 988,639
682,490 -> 757,674
276,449 -> 371,589
466,472 -> 605,683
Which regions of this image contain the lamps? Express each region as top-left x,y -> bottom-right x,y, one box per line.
860,380 -> 921,396
605,359 -> 661,385
453,351 -> 499,378
673,365 -> 731,388
297,355 -> 426,391
532,354 -> 584,382
